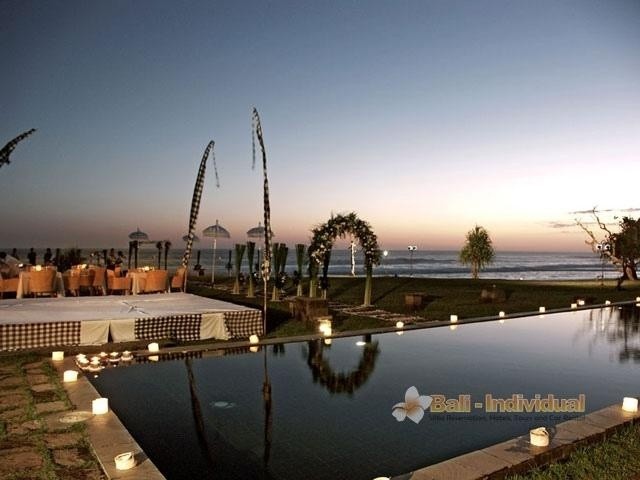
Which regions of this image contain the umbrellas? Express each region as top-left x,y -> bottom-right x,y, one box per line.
202,219 -> 265,283
128,227 -> 148,268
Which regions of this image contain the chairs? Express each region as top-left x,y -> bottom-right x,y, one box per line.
0,263 -> 187,299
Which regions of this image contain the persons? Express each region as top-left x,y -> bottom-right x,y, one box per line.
0,248 -> 126,274
